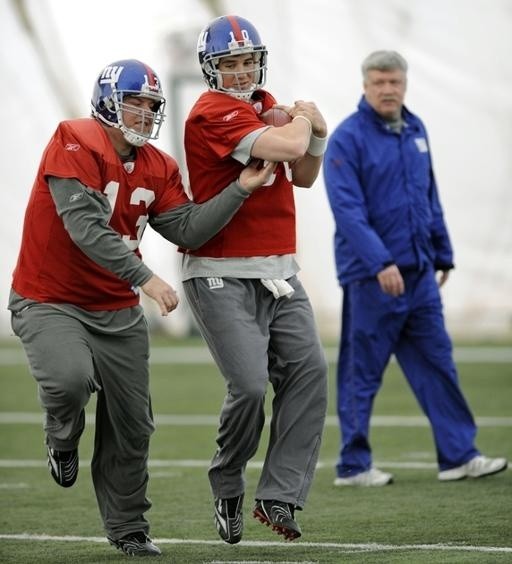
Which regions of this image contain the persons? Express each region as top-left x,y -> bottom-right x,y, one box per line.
6,54 -> 279,560
321,46 -> 509,488
179,11 -> 331,546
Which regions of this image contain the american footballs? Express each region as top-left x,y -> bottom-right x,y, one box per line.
258,107 -> 294,128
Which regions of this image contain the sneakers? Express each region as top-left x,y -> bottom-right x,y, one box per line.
334,468 -> 393,487
106,531 -> 162,556
46,446 -> 78,488
214,493 -> 244,544
253,500 -> 302,541
438,454 -> 508,481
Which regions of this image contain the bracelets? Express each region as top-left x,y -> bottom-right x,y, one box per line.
305,133 -> 328,157
291,114 -> 313,133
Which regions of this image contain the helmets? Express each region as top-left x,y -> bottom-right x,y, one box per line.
89,58 -> 166,147
197,14 -> 268,101
